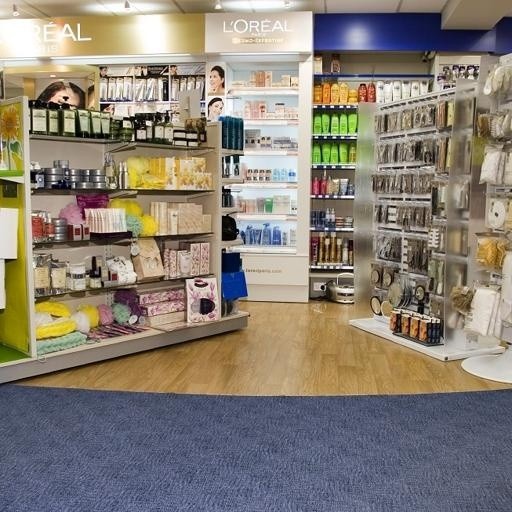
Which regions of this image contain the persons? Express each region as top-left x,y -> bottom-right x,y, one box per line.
101,104 -> 116,115
208,66 -> 226,95
170,64 -> 179,77
36,82 -> 86,110
99,66 -> 110,78
208,98 -> 223,119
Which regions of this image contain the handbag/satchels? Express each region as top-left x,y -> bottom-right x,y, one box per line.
187,278 -> 222,324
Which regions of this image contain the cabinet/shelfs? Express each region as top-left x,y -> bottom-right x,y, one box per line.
1,53 -> 511,383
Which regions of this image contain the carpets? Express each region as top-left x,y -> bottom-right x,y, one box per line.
1,385 -> 512,512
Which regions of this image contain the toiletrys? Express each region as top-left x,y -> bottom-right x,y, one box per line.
118,161 -> 130,189
217,69 -> 300,247
310,170 -> 354,230
